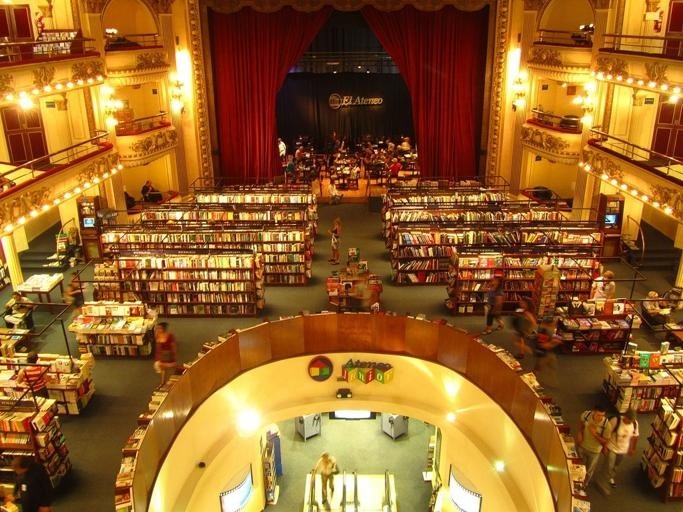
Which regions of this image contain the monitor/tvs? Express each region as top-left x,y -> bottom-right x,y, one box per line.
604,214 -> 617,228
82,216 -> 96,229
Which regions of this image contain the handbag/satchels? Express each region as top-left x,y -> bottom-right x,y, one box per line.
330,232 -> 341,250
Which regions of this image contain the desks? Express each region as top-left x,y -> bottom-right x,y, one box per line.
17,275 -> 65,315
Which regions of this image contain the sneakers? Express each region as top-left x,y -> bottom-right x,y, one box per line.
608,477 -> 617,488
327,257 -> 340,265
480,323 -> 504,335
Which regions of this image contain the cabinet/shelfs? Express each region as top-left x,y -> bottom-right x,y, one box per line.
445,248 -> 636,354
70,254 -> 262,358
0,300 -> 96,509
602,295 -> 682,504
98,181 -> 315,286
380,177 -> 601,282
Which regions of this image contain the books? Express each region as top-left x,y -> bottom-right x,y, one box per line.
0,328 -> 94,512
603,342 -> 682,500
321,310 -> 590,512
328,246 -> 382,311
115,310 -> 309,512
67,181 -> 319,356
380,183 -> 643,354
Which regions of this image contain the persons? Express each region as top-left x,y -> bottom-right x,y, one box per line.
141,180 -> 160,202
277,130 -> 418,205
